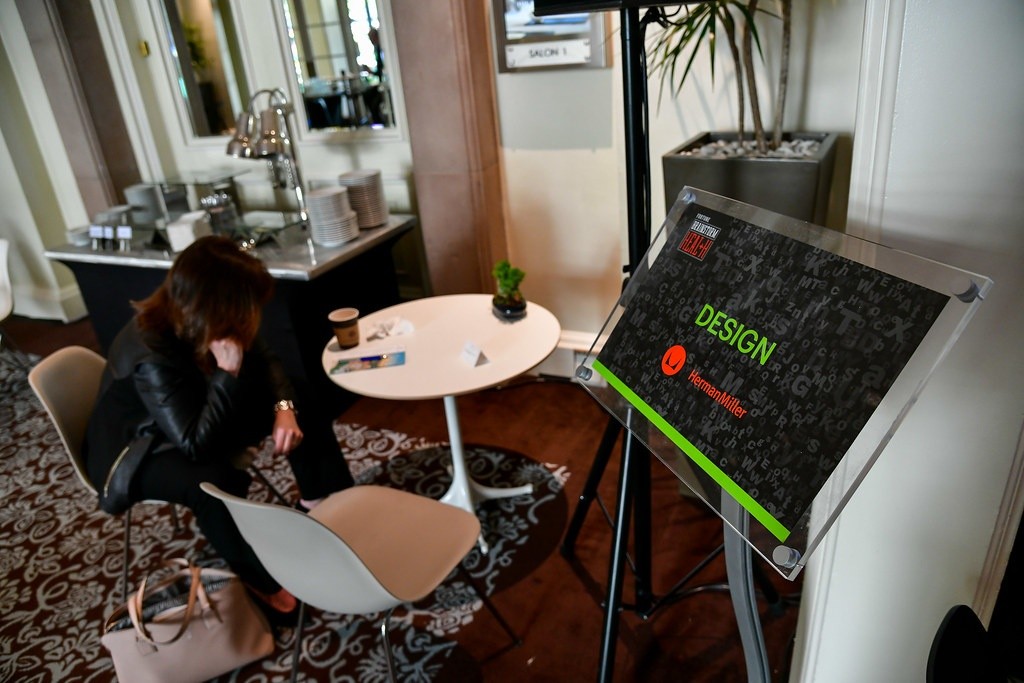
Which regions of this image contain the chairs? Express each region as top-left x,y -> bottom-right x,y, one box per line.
197,480 -> 522,682
0,239 -> 31,385
28,345 -> 289,604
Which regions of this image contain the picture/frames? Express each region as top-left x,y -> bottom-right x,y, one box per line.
490,1 -> 615,74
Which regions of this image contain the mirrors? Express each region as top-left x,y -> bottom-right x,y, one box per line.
157,0 -> 396,140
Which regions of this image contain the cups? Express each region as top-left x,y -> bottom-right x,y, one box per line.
327,307 -> 360,349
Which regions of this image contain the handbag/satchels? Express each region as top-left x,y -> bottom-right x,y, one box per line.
101,558 -> 275,683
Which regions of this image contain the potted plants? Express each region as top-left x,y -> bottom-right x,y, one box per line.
491,259 -> 528,325
602,0 -> 838,227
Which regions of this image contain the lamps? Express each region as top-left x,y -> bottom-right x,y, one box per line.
226,88 -> 309,229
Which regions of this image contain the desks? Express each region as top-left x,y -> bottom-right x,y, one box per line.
322,293 -> 561,555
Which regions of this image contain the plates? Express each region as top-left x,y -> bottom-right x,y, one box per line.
305,168 -> 390,248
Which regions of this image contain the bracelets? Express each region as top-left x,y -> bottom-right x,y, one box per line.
274,400 -> 293,411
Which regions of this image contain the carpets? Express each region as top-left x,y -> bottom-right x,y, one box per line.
0,345 -> 571,683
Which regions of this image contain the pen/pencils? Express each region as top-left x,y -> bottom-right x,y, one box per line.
339,355 -> 388,365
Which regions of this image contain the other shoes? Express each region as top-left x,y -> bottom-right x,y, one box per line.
295,494 -> 328,514
245,585 -> 313,625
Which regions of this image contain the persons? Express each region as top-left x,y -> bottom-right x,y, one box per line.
84,236 -> 355,630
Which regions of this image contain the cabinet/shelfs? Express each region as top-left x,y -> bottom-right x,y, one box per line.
42,212 -> 425,421
